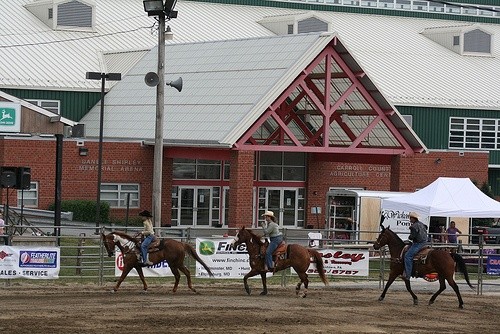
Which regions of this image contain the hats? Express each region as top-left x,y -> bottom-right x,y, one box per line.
139,210 -> 153,217
261,211 -> 276,219
406,211 -> 419,219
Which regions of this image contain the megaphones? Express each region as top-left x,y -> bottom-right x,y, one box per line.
144,72 -> 160,87
166,76 -> 183,92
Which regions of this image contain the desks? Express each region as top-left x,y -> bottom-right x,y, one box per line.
419,244 -> 500,266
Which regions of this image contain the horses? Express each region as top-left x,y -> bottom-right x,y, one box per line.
230,225 -> 329,298
373,225 -> 476,310
101,231 -> 214,294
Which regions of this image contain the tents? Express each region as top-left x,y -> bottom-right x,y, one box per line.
381,176 -> 499,222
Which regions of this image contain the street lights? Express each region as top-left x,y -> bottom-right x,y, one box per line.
143,0 -> 177,240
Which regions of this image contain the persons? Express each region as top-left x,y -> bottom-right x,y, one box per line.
438,224 -> 447,244
0,212 -> 6,236
260,209 -> 285,270
449,220 -> 463,248
399,212 -> 429,280
138,210 -> 157,268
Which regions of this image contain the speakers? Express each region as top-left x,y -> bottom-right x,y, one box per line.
0,166 -> 31,190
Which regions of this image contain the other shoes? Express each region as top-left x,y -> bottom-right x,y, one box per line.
399,275 -> 409,280
138,260 -> 146,264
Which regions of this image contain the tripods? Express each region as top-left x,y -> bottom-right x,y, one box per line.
2,187 -> 47,236
